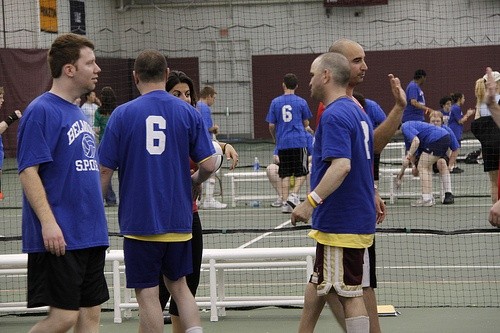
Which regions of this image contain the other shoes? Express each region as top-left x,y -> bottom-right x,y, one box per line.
287,193 -> 301,209
451,166 -> 461,173
203,199 -> 228,208
272,197 -> 283,206
443,192 -> 455,204
281,202 -> 294,212
410,196 -> 436,207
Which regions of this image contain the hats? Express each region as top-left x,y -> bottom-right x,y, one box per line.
484,71 -> 500,84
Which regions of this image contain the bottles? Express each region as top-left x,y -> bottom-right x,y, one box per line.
245,201 -> 263,207
253,157 -> 259,170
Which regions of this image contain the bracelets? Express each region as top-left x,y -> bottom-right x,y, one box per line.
222,142 -> 228,152
308,193 -> 317,208
4,111 -> 19,127
310,190 -> 323,204
425,107 -> 429,115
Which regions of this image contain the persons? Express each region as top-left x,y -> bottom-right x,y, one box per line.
81,92 -> 102,129
93,86 -> 119,208
447,92 -> 475,175
96,48 -> 216,333
195,86 -> 238,211
160,70 -> 204,333
401,69 -> 460,204
471,67 -> 500,229
15,34 -> 110,333
393,121 -> 451,207
265,39 -> 407,333
0,85 -> 23,193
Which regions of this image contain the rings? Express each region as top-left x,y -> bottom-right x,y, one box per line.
237,159 -> 239,161
